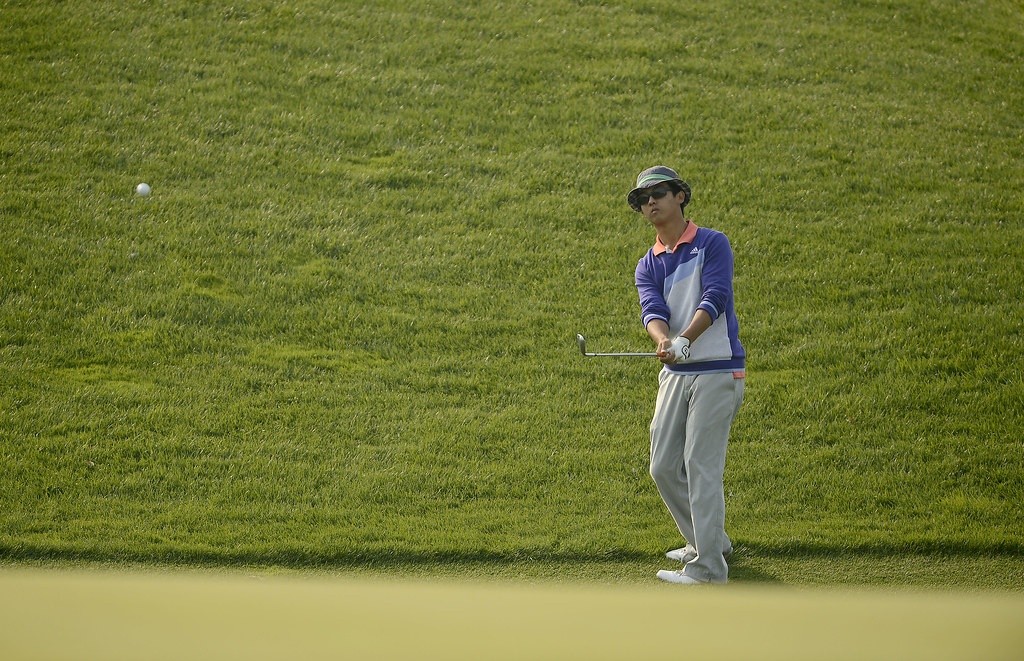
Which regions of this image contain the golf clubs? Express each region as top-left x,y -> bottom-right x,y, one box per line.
576,333 -> 690,357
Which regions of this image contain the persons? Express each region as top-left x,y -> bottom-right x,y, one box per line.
628,166 -> 745,586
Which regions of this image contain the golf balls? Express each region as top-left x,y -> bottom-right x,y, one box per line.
136,183 -> 152,197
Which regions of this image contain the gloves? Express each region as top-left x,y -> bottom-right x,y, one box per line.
664,335 -> 691,364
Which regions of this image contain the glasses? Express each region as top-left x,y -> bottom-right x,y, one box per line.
636,185 -> 673,205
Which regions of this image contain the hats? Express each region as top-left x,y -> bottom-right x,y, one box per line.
627,166 -> 691,214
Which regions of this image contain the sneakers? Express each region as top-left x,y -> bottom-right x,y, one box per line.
656,570 -> 706,586
666,544 -> 734,562
